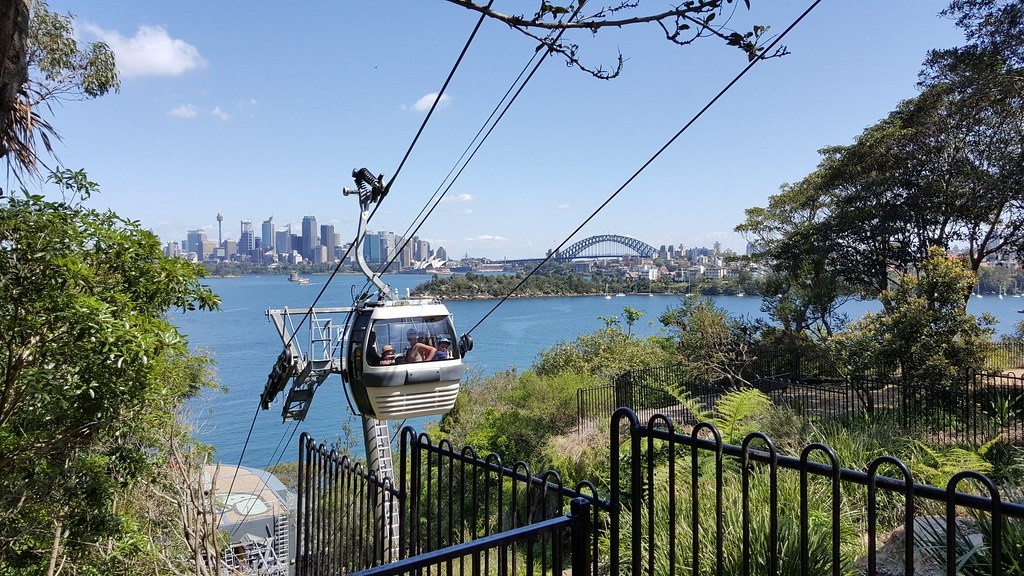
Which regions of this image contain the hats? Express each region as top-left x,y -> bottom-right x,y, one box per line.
381,345 -> 396,354
437,338 -> 451,345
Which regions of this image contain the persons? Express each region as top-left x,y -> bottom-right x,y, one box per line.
365,329 -> 452,366
